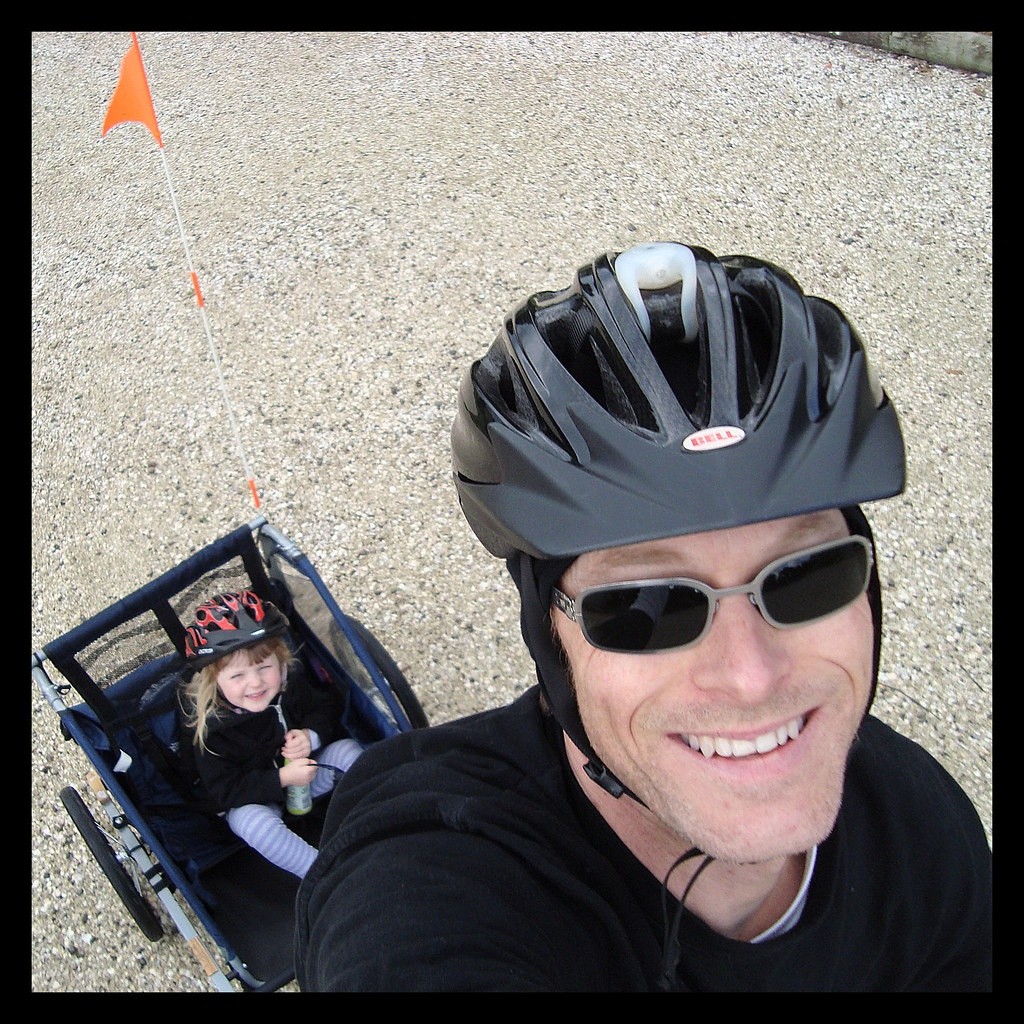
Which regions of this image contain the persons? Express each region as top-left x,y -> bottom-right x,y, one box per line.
176,589 -> 367,881
296,241 -> 992,995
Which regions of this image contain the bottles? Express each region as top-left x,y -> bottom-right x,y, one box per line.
284,753 -> 312,815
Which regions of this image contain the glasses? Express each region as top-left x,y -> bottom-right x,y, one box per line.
550,534 -> 874,655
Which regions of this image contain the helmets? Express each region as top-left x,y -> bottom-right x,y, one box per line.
185,591 -> 289,674
452,243 -> 907,558
